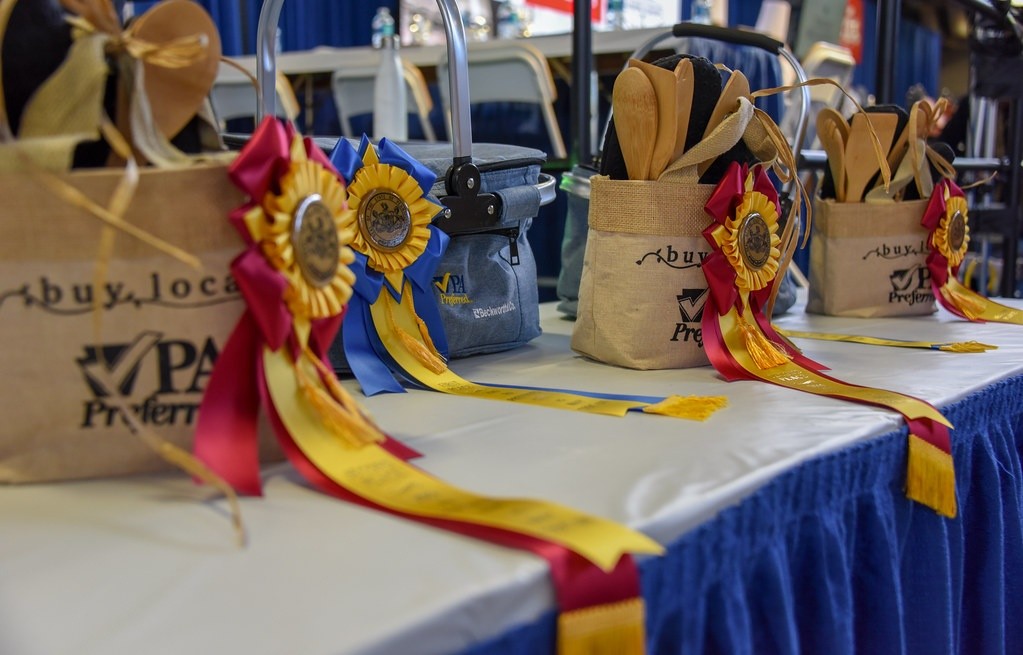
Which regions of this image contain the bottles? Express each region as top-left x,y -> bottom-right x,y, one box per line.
371,34 -> 410,143
692,0 -> 713,27
497,0 -> 517,40
607,1 -> 624,29
371,7 -> 395,49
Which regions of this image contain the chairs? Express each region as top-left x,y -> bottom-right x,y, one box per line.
333,64 -> 435,149
397,44 -> 574,300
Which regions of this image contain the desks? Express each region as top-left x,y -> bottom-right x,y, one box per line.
4,294 -> 1023,650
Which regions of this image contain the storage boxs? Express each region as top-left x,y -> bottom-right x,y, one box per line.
551,22 -> 809,326
249,0 -> 560,361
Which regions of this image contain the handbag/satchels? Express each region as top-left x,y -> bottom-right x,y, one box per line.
1,1 -> 265,480
253,1 -> 558,361
566,24 -> 807,371
803,104 -> 973,319
